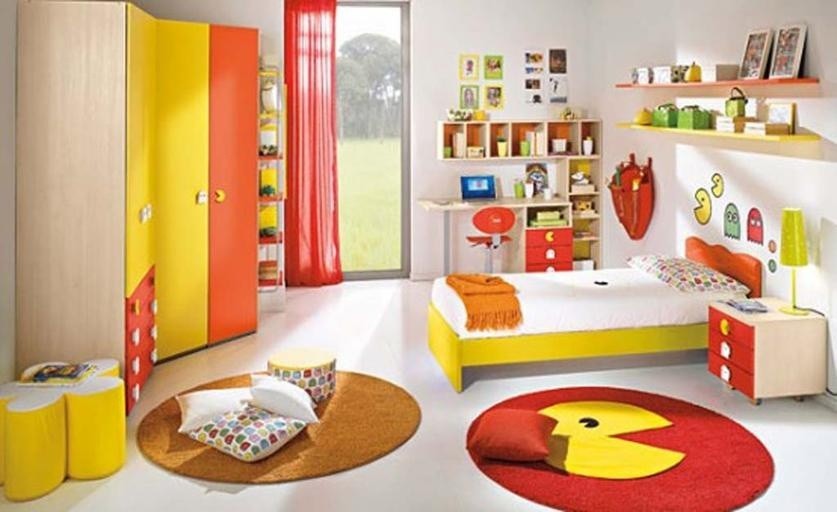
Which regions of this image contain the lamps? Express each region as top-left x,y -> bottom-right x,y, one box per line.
778,208 -> 809,316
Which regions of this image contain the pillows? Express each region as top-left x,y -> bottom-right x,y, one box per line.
175,374 -> 321,463
471,408 -> 558,462
626,256 -> 749,297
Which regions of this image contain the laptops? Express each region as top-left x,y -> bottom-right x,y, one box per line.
460,175 -> 508,207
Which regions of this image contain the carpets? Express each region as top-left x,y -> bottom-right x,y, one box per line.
468,387 -> 773,511
136,371 -> 421,484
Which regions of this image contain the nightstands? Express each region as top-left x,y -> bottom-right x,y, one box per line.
708,296 -> 827,406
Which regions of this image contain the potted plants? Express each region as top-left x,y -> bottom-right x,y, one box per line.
494,135 -> 592,158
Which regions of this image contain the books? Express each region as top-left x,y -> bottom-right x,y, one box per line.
16,363 -> 98,388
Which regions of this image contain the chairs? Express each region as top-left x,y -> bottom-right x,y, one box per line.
467,207 -> 516,275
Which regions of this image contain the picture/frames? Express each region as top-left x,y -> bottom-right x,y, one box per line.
525,49 -> 567,104
459,50 -> 504,109
738,24 -> 806,79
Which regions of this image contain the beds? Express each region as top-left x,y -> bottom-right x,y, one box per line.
429,237 -> 761,391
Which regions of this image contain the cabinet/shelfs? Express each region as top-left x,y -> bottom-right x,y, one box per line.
17,1 -> 160,416
421,119 -> 604,273
615,78 -> 820,142
157,17 -> 259,360
259,61 -> 289,313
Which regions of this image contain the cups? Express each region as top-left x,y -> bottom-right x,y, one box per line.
519,141 -> 530,157
444,146 -> 452,158
543,187 -> 554,202
524,180 -> 533,199
583,140 -> 593,157
513,179 -> 525,199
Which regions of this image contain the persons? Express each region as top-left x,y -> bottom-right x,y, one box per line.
465,60 -> 474,76
550,50 -> 566,72
464,88 -> 475,105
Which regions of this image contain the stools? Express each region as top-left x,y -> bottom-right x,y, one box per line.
269,349 -> 336,403
0,359 -> 125,497
